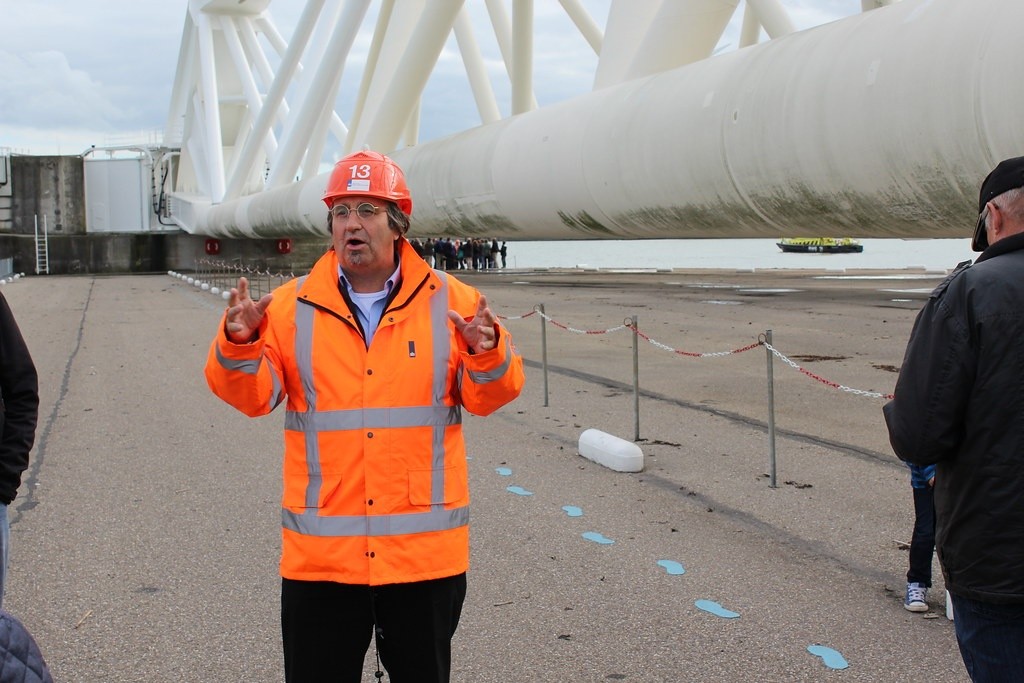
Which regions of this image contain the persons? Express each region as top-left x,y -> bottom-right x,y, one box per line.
204,149 -> 526,683
883,156 -> 1024,683
0,290 -> 39,608
410,237 -> 507,271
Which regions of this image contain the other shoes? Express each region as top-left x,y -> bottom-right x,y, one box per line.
460,268 -> 465,269
480,268 -> 485,270
501,267 -> 506,268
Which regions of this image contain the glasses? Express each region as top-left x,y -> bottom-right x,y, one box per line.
327,203 -> 378,220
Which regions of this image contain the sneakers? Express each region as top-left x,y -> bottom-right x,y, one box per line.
904,582 -> 928,611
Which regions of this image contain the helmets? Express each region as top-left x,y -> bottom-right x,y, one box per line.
322,150 -> 412,217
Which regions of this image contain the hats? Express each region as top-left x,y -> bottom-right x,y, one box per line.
456,240 -> 460,242
972,157 -> 1024,251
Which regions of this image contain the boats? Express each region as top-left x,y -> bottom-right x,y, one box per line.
776,236 -> 863,253
